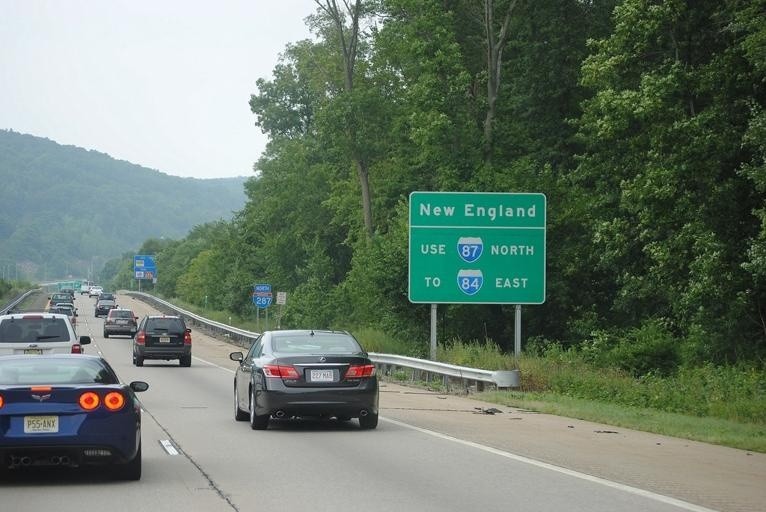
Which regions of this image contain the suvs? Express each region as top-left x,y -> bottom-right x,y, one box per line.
0,311 -> 92,355
132,313 -> 194,369
102,308 -> 138,341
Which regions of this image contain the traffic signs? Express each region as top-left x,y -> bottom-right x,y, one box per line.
407,190 -> 545,304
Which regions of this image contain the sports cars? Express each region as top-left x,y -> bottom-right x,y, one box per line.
0,355 -> 149,480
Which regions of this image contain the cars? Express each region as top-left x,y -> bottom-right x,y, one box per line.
226,328 -> 381,430
45,279 -> 116,328
